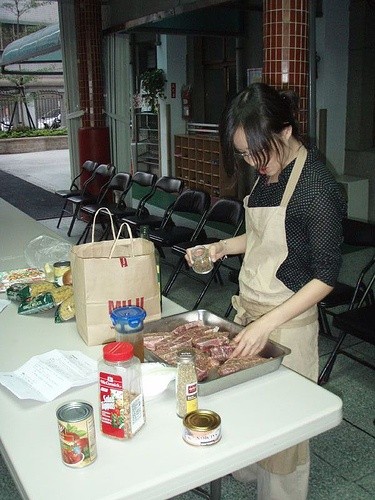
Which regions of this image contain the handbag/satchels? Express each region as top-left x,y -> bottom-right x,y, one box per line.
71,207 -> 161,346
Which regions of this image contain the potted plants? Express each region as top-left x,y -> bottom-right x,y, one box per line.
138,67 -> 169,115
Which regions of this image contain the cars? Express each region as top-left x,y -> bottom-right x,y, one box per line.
37,108 -> 61,130
0,120 -> 13,132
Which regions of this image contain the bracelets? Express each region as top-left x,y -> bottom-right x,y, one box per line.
217,239 -> 228,262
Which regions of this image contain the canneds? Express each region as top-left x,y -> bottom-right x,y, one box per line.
182,409 -> 223,445
191,245 -> 214,274
56,402 -> 97,468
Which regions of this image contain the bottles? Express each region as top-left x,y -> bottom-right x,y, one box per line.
97,341 -> 145,441
176,352 -> 198,419
193,247 -> 213,273
138,224 -> 162,315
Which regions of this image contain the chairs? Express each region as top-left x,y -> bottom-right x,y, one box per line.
318,217 -> 375,341
54,160 -> 245,318
318,272 -> 375,424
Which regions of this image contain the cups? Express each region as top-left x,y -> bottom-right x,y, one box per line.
110,305 -> 146,364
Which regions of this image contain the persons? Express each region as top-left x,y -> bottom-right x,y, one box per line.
185,81 -> 349,500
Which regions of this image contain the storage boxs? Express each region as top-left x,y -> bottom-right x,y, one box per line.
143,308 -> 293,397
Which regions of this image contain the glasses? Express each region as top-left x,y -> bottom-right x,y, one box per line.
232,147 -> 264,162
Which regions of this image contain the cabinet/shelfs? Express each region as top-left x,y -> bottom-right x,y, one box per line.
174,134 -> 238,200
132,106 -> 162,179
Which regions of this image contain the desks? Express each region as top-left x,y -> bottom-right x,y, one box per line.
0,196 -> 343,500
